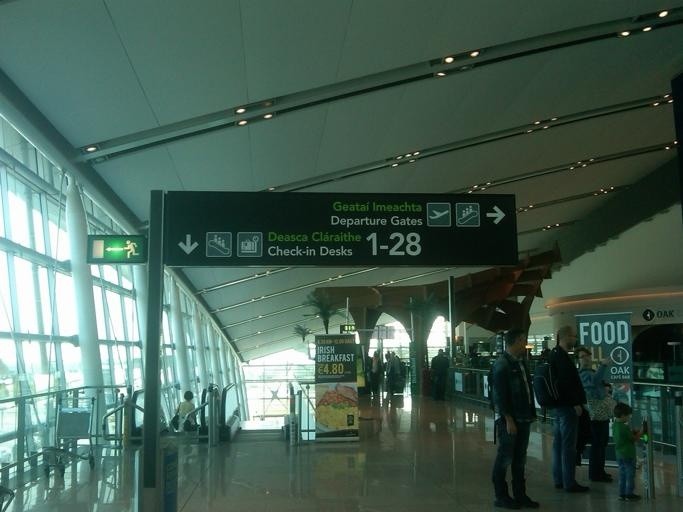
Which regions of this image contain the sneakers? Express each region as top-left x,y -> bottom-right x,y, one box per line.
616,492 -> 643,502
588,473 -> 613,484
564,482 -> 590,492
554,484 -> 564,488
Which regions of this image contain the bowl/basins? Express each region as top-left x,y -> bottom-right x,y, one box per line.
543,337 -> 551,340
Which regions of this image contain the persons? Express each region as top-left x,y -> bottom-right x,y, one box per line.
175,391 -> 198,432
576,347 -> 613,483
546,326 -> 590,493
491,329 -> 542,508
611,402 -> 643,501
430,349 -> 450,400
371,350 -> 401,393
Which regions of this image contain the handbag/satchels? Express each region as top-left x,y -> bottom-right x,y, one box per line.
585,391 -> 622,422
171,415 -> 181,430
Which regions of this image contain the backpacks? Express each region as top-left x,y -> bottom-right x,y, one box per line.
533,361 -> 563,407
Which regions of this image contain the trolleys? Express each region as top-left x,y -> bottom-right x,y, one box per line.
38,396 -> 96,477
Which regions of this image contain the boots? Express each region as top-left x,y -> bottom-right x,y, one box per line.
510,478 -> 540,508
492,476 -> 512,508
